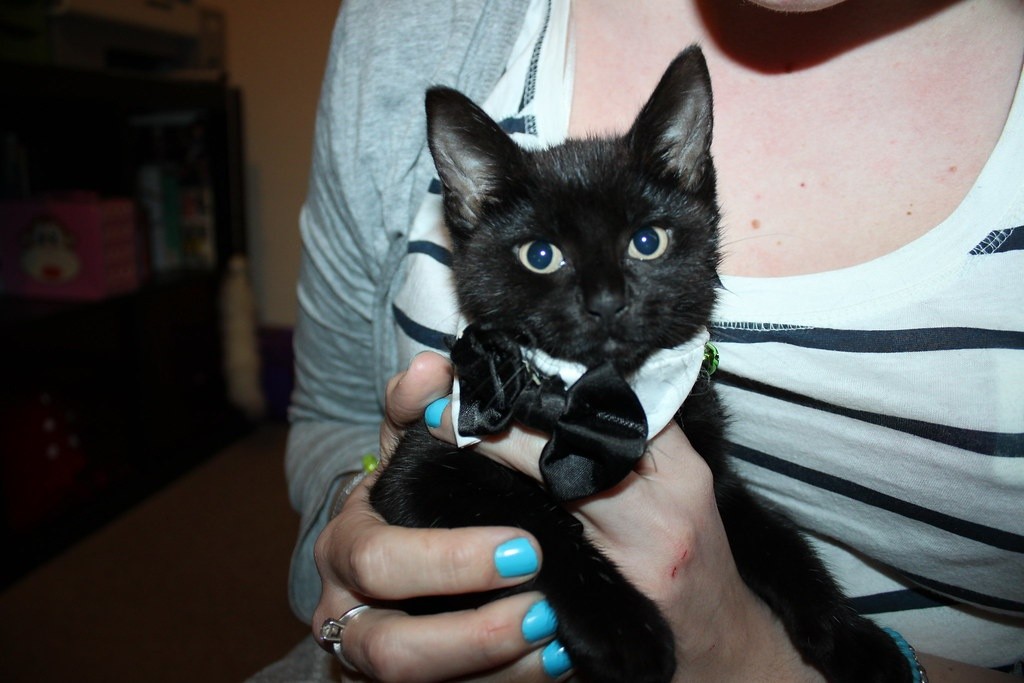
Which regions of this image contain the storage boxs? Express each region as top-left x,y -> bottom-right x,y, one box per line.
0,0 -> 227,88
0,192 -> 136,305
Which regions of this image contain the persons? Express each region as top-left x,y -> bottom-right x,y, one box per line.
245,1 -> 1024,683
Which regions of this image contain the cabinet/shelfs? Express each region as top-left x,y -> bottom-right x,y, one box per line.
0,71 -> 248,595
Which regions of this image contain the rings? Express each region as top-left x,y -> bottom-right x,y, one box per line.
319,603 -> 385,675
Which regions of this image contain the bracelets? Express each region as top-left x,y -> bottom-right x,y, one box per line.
884,627 -> 928,683
332,455 -> 379,519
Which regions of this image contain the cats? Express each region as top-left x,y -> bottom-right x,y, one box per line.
369,40 -> 915,683
